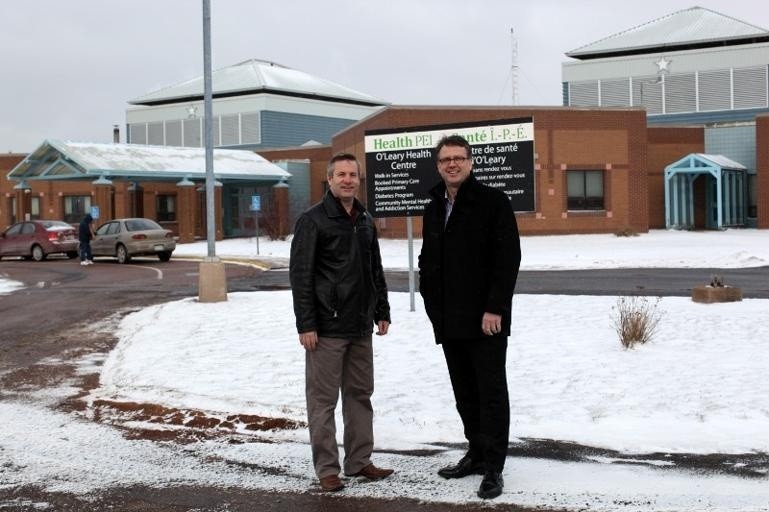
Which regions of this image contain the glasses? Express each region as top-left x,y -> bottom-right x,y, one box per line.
438,157 -> 469,166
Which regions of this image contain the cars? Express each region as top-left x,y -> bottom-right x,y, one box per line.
76,218 -> 175,265
0,218 -> 79,263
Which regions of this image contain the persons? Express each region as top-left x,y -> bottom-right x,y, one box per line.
415,136 -> 524,499
288,153 -> 395,492
78,215 -> 96,266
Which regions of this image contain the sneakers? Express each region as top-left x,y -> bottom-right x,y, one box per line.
80,259 -> 93,265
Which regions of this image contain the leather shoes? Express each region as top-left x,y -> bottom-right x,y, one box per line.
438,456 -> 484,478
360,463 -> 393,479
320,474 -> 344,491
479,470 -> 502,498
